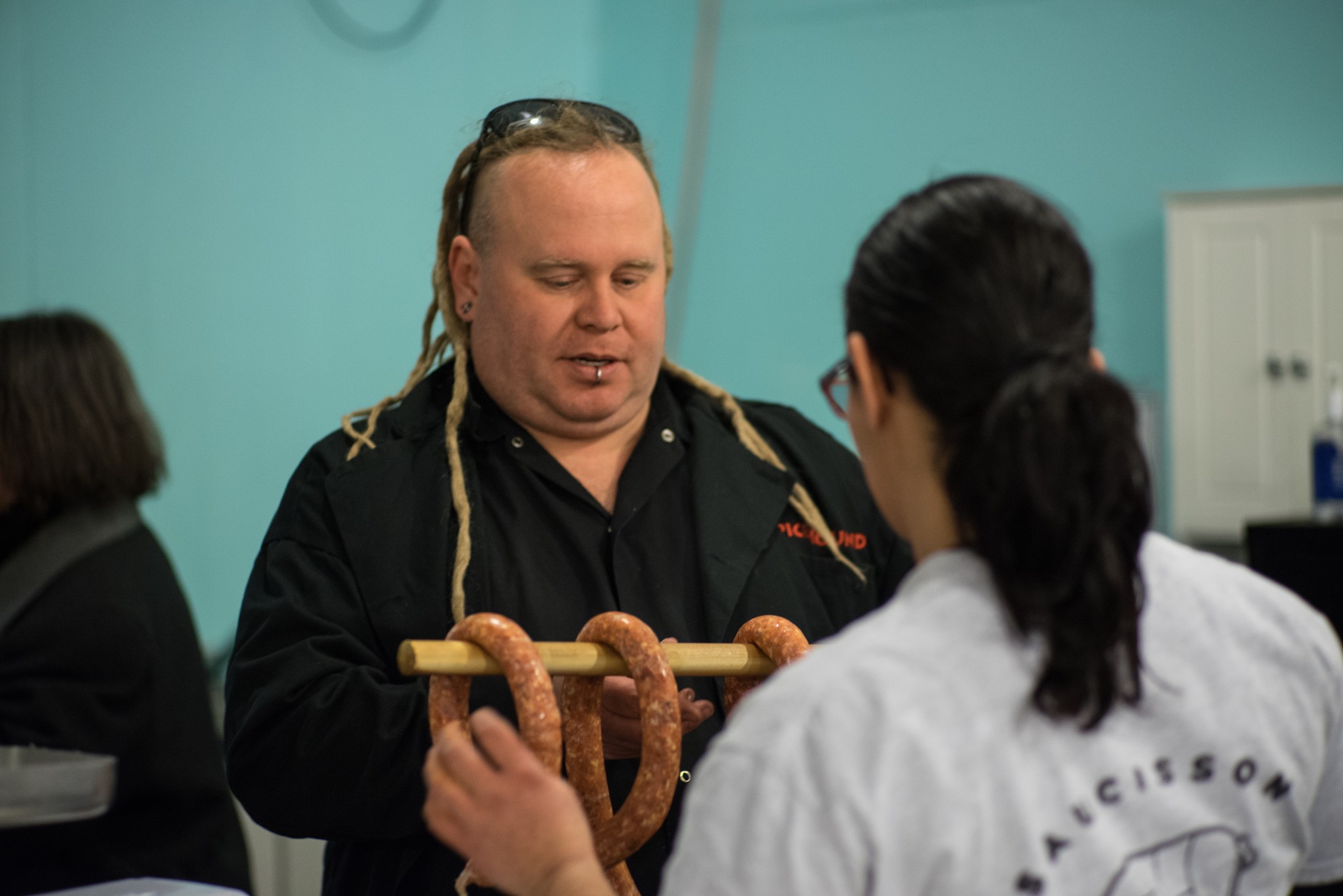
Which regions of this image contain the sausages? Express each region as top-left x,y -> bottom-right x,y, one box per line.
427,611 -> 813,895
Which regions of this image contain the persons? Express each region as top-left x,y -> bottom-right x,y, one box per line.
0,305 -> 254,896
221,97 -> 921,896
416,171 -> 1342,896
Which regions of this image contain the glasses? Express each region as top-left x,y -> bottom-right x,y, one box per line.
457,100 -> 643,244
